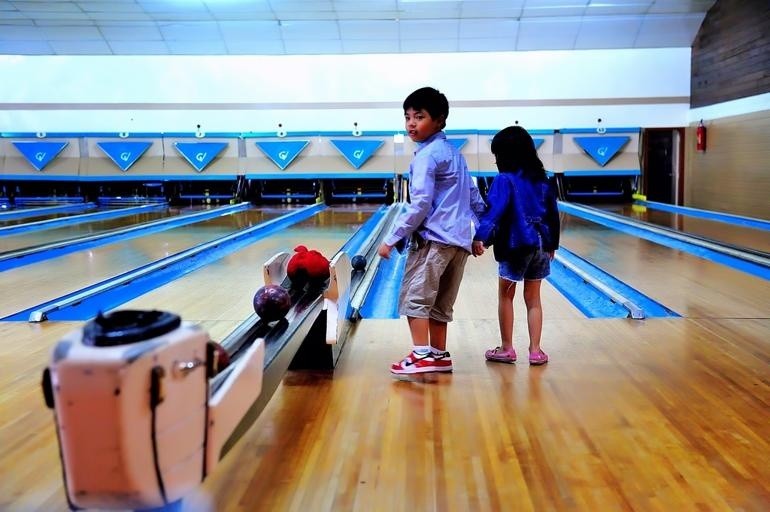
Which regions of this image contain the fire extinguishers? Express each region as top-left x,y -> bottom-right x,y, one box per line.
696,119 -> 706,153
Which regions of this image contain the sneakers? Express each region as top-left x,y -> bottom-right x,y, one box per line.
485,347 -> 517,362
528,350 -> 548,365
390,351 -> 452,374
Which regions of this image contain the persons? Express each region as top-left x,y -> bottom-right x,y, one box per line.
471,127 -> 559,365
378,87 -> 486,375
